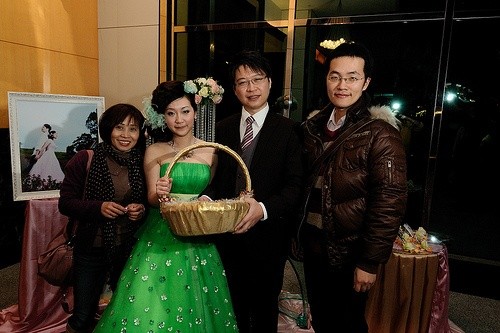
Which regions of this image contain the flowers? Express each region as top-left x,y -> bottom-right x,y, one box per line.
183,76 -> 224,105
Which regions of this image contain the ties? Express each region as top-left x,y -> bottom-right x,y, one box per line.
241,116 -> 255,167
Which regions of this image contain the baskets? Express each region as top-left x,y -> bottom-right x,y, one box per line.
160,142 -> 252,236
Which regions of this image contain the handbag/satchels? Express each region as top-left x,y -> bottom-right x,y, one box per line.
38,234 -> 74,285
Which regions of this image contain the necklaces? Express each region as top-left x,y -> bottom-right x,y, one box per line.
169,137 -> 196,159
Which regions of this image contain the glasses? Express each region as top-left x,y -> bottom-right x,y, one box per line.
327,75 -> 365,84
235,75 -> 267,87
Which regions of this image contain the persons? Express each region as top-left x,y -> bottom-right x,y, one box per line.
197,52 -> 305,333
93,78 -> 239,333
58,103 -> 148,333
28,123 -> 65,184
293,42 -> 408,333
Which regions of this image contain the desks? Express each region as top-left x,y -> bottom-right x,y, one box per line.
0,199 -> 116,333
366,231 -> 465,333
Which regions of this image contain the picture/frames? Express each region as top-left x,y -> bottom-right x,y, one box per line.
7,91 -> 106,202
